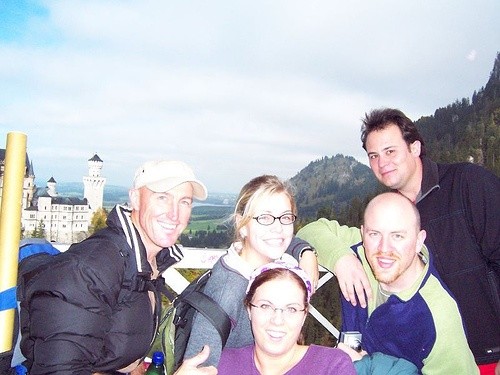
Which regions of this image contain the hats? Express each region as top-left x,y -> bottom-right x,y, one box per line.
133,159 -> 208,200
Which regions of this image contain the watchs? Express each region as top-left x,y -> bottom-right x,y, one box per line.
300,248 -> 318,259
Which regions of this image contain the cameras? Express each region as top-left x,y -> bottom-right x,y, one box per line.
340,331 -> 363,353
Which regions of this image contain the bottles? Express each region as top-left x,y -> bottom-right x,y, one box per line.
145,351 -> 164,375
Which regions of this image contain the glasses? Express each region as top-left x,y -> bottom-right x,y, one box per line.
243,213 -> 298,226
249,303 -> 305,316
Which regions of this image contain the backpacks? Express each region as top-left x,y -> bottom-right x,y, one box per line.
9,229 -> 174,375
146,269 -> 232,375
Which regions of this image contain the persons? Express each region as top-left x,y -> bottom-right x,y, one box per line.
294,192 -> 480,375
361,108 -> 500,374
216,260 -> 359,375
181,174 -> 320,370
18,159 -> 219,375
